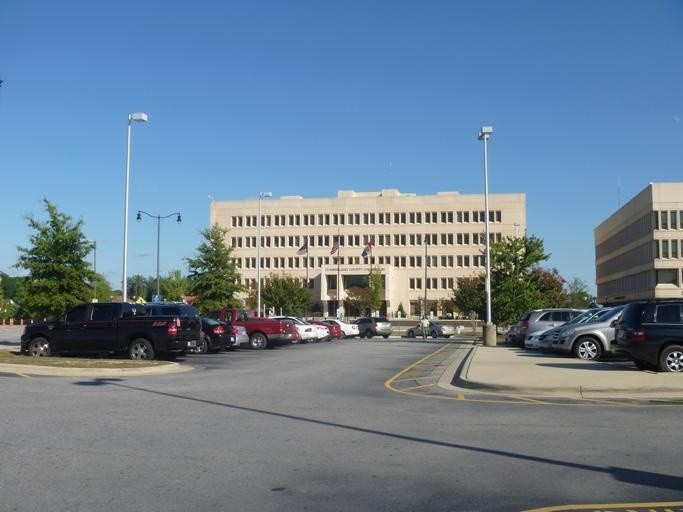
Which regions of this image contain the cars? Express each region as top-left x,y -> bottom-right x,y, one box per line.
271,315 -> 330,343
200,316 -> 240,354
400,320 -> 457,339
351,317 -> 394,340
320,319 -> 361,340
304,319 -> 341,340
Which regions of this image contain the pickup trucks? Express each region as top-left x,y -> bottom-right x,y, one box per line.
216,306 -> 297,350
19,301 -> 203,362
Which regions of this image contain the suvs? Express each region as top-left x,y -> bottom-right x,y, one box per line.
503,297 -> 683,373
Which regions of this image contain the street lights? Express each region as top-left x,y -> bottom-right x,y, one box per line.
257,191 -> 273,318
512,221 -> 521,236
121,110 -> 149,305
476,123 -> 498,347
135,209 -> 185,301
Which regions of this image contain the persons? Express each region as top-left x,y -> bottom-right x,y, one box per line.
419,315 -> 430,340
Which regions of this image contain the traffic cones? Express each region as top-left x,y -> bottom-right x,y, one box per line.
2,318 -> 47,328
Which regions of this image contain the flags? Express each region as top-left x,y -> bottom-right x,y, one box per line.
296,244 -> 308,255
330,236 -> 338,254
361,241 -> 370,256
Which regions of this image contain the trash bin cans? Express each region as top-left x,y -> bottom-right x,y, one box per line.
482,324 -> 497,347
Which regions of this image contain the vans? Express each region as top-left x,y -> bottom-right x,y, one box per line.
129,301 -> 203,355
